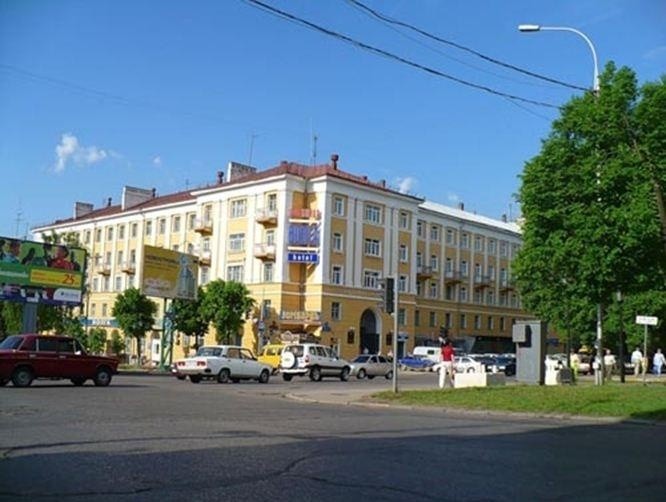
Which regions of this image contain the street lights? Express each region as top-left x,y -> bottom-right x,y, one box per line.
519,24 -> 604,385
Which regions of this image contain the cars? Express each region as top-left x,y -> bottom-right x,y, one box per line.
173,343 -> 394,383
0,333 -> 119,388
397,346 -> 637,375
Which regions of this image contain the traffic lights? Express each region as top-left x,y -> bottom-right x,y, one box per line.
375,278 -> 394,314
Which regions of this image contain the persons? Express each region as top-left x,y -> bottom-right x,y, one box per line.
438,336 -> 455,389
570,346 -> 666,382
